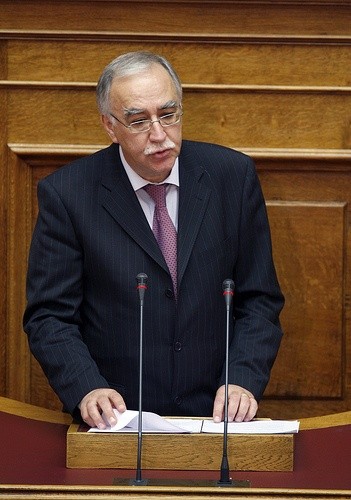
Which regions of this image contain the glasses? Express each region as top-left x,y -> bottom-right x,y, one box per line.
107,102 -> 184,135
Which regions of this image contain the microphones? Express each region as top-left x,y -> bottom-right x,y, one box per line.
134,272 -> 148,483
220,279 -> 234,484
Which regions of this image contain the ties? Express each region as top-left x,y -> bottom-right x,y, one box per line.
145,182 -> 180,303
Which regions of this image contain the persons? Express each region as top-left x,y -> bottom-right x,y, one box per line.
23,53 -> 285,429
